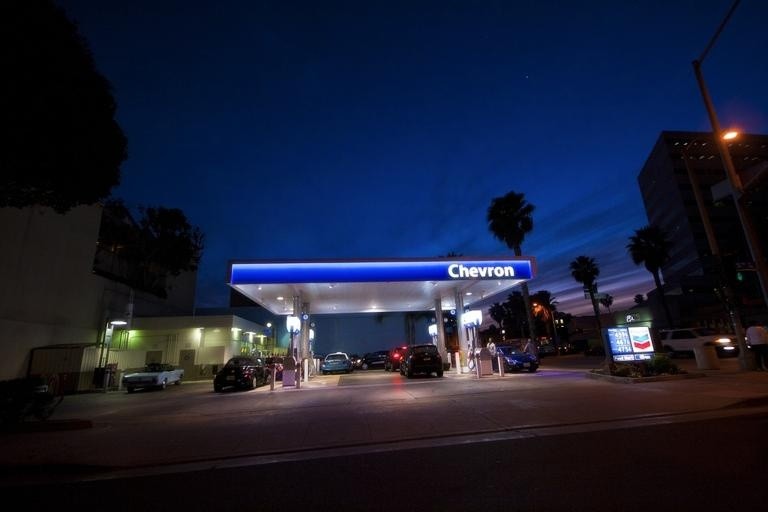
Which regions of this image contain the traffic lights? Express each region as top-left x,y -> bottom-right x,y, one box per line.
737,272 -> 744,282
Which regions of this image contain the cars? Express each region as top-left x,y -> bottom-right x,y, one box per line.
120,361 -> 187,394
504,336 -> 578,355
382,345 -> 407,372
211,354 -> 271,391
266,349 -> 391,379
491,343 -> 539,374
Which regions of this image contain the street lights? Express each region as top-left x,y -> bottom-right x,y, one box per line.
99,316 -> 128,372
679,123 -> 754,372
532,300 -> 559,341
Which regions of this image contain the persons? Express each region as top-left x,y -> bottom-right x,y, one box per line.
746,326 -> 768,371
487,337 -> 496,358
524,339 -> 535,356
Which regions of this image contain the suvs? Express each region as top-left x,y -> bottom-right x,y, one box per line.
656,327 -> 740,359
399,343 -> 445,377
